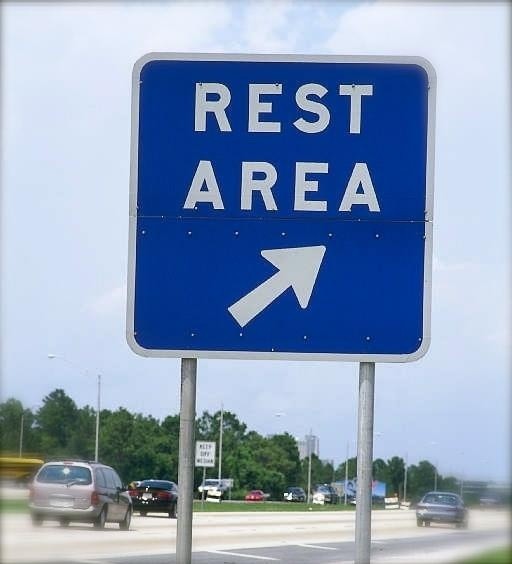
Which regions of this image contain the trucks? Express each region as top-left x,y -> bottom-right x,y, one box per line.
198,479 -> 235,500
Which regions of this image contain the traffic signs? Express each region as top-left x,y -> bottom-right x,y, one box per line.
125,52 -> 436,365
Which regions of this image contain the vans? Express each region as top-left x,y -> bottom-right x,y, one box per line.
27,461 -> 132,531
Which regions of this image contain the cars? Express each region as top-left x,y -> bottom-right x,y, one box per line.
312,484 -> 338,503
480,492 -> 511,512
128,480 -> 178,518
284,487 -> 306,502
350,495 -> 356,505
416,491 -> 470,530
245,490 -> 265,501
15,473 -> 35,489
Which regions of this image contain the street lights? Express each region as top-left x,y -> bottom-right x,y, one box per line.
345,431 -> 383,507
19,403 -> 40,458
460,459 -> 489,499
48,354 -> 102,462
403,441 -> 439,507
275,413 -> 312,503
